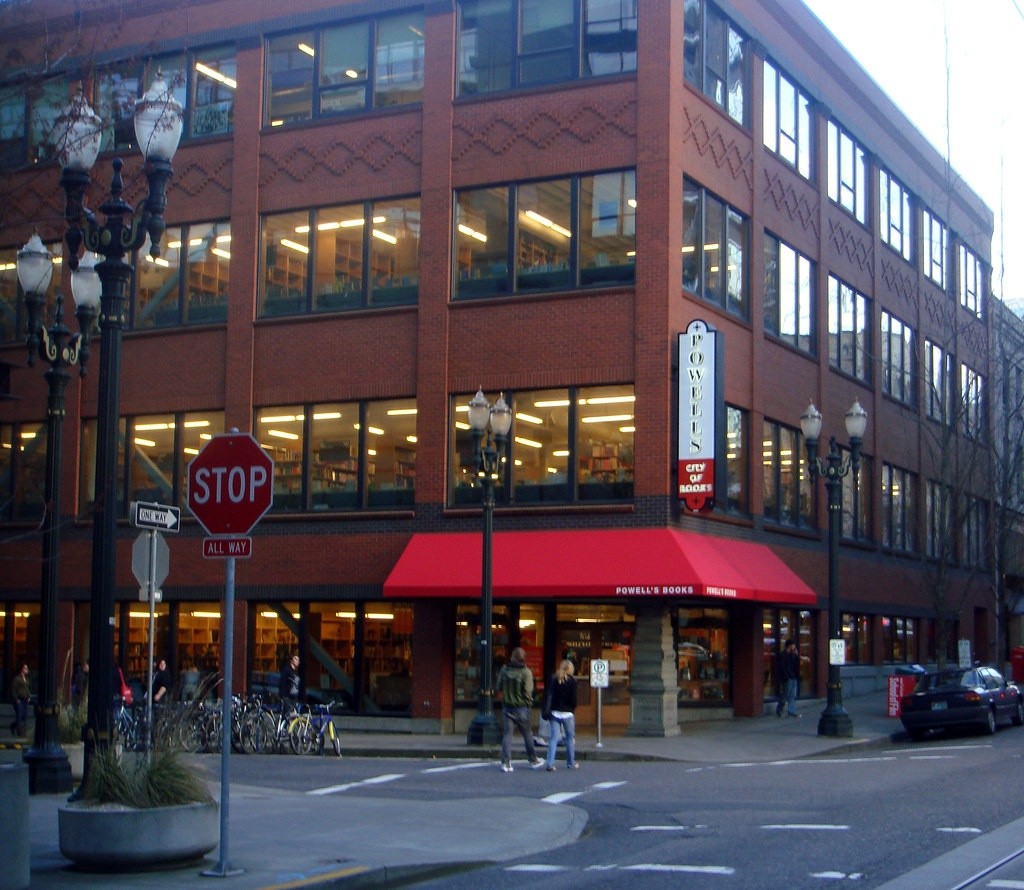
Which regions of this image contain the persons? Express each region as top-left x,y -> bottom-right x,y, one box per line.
774,638 -> 809,719
72,659 -> 89,717
110,663 -> 133,728
9,664 -> 31,737
541,660 -> 580,772
143,658 -> 167,749
177,643 -> 219,699
278,654 -> 304,722
495,647 -> 545,773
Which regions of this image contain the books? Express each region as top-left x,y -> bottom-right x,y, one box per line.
393,452 -> 417,489
267,446 -> 376,492
590,444 -> 633,484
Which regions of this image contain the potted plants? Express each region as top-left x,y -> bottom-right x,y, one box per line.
57,671 -> 223,869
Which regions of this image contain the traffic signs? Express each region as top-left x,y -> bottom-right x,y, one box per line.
136,502 -> 181,535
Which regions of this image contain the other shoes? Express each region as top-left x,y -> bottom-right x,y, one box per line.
776,710 -> 783,716
567,761 -> 579,769
788,710 -> 803,719
547,766 -> 556,772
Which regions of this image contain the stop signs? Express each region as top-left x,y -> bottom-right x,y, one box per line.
185,432 -> 275,537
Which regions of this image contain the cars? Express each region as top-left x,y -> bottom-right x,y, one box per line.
252,671 -> 327,708
782,630 -> 810,656
899,663 -> 1024,741
679,643 -> 709,661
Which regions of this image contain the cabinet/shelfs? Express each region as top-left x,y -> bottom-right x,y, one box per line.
257,612 -> 299,680
305,611 -> 405,704
315,230 -> 390,292
376,437 -> 414,488
114,616 -> 157,675
267,254 -> 306,297
154,616 -> 220,671
395,234 -> 485,288
587,456 -> 635,483
486,227 -> 559,272
273,460 -> 356,491
188,249 -> 229,299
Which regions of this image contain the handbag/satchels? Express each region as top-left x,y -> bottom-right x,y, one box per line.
539,712 -> 565,741
541,691 -> 554,720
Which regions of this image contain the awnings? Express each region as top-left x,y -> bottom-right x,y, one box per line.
382,529 -> 818,605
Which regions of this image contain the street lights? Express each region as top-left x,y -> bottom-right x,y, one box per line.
50,73 -> 186,807
799,395 -> 868,737
468,384 -> 513,747
17,227 -> 103,798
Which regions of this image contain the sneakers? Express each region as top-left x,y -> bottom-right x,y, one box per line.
529,757 -> 545,769
500,761 -> 514,773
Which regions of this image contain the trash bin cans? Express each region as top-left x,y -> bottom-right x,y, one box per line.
888,665 -> 927,717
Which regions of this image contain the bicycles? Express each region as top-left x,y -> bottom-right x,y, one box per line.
113,690 -> 344,757
